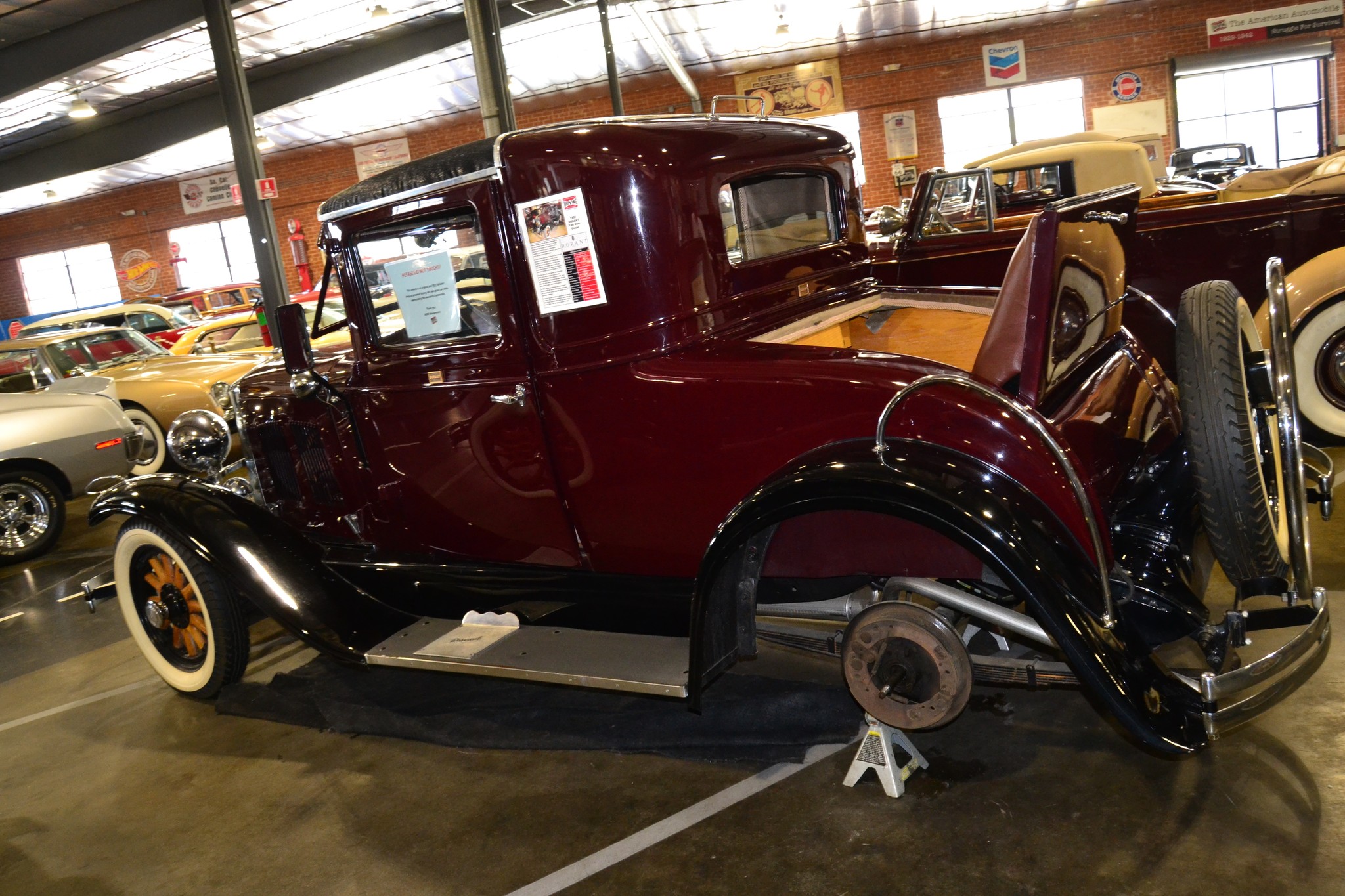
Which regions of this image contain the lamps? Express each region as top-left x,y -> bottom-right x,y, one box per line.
69,89 -> 97,118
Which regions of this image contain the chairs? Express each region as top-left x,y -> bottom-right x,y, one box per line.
46,345 -> 79,376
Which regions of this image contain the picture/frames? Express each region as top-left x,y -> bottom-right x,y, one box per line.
893,165 -> 918,187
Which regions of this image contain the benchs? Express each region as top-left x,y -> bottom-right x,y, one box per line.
0,373 -> 33,393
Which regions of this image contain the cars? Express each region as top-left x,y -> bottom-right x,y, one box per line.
81,94 -> 1339,763
0,242 -> 497,564
861,131 -> 1345,449
